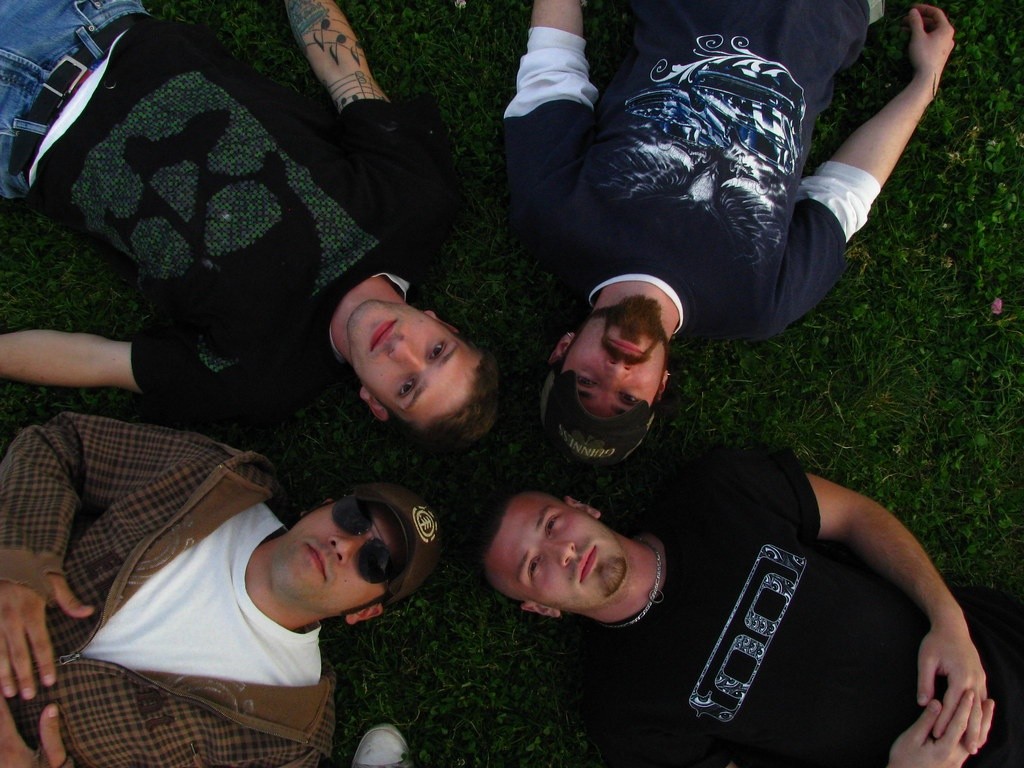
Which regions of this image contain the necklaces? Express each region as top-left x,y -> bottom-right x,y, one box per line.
595,533 -> 665,629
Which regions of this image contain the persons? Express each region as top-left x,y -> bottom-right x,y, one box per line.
0,410 -> 443,768
0,0 -> 501,453
474,444 -> 1023,768
502,0 -> 957,467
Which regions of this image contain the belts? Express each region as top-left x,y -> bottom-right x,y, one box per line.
9,13 -> 158,176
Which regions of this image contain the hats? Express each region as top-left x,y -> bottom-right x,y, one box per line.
347,482 -> 441,607
537,359 -> 655,473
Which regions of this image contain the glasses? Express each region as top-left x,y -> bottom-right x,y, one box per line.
332,496 -> 401,603
